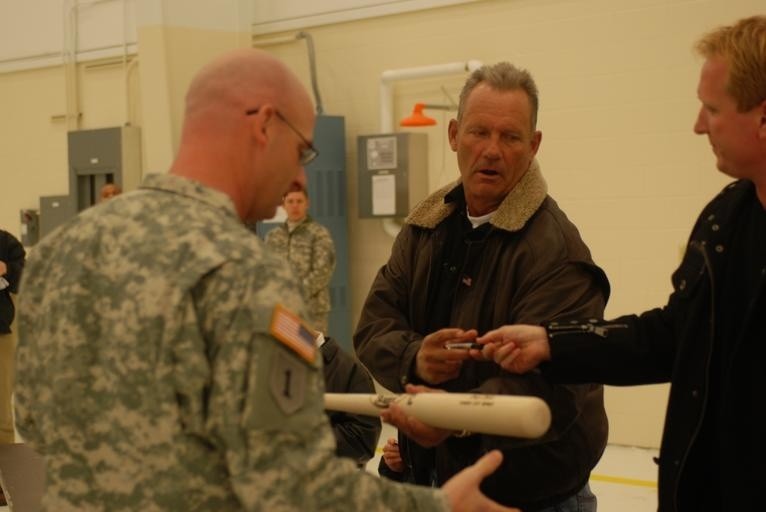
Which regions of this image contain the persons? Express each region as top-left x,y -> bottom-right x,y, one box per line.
316,330 -> 380,469
0,228 -> 27,506
466,12 -> 766,512
11,53 -> 526,512
264,189 -> 336,338
352,62 -> 612,512
99,183 -> 120,201
376,438 -> 409,482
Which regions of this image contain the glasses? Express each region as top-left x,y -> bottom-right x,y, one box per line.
248,102 -> 318,164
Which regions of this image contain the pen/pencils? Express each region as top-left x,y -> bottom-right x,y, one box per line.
445,343 -> 485,351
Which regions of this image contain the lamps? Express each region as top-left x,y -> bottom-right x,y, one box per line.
399,100 -> 456,129
